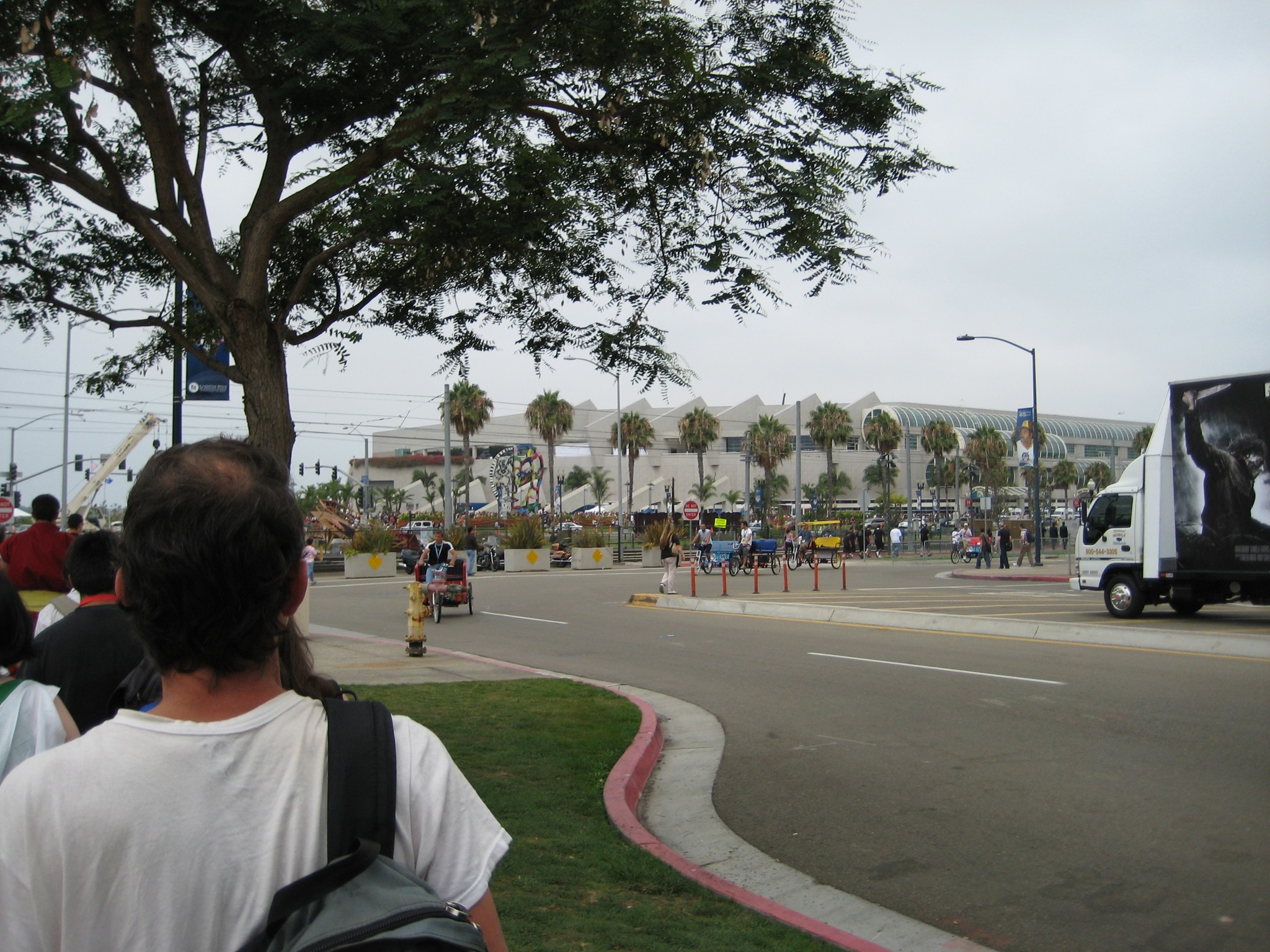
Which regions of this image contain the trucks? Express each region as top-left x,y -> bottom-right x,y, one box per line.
1070,372 -> 1270,619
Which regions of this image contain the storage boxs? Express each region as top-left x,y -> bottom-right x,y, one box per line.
552,551 -> 564,557
551,542 -> 561,550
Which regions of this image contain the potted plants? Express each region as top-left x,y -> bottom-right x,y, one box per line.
498,512 -> 551,572
567,526 -> 613,569
426,518 -> 477,575
338,515 -> 398,578
633,517 -> 685,567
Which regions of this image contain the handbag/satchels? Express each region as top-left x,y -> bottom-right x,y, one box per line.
669,535 -> 678,556
986,543 -> 991,553
1006,542 -> 1013,552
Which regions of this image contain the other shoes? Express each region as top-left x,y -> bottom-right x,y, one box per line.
708,562 -> 712,568
740,566 -> 746,570
920,555 -> 923,557
660,583 -> 664,593
928,554 -> 932,557
797,563 -> 801,567
668,590 -> 677,594
848,552 -> 882,559
1013,562 -> 1019,567
975,566 -> 980,569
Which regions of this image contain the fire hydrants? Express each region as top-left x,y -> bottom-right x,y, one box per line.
403,571 -> 471,658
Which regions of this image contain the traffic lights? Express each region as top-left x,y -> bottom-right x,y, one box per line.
10,464 -> 19,480
315,462 -> 321,475
14,491 -> 22,508
1,483 -> 9,496
332,466 -> 337,480
299,463 -> 304,475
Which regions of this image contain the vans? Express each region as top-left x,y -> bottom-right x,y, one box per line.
401,521 -> 433,533
999,505 -> 1087,526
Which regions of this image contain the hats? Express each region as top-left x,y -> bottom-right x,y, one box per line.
964,523 -> 968,526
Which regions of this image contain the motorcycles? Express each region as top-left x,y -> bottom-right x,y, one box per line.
552,542 -> 572,567
475,536 -> 500,571
398,538 -> 429,575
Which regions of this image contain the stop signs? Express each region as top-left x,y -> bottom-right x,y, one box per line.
683,499 -> 700,520
0,498 -> 14,523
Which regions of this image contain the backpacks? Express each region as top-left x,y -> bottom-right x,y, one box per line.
110,621 -> 164,712
1022,529 -> 1033,543
242,702 -> 487,952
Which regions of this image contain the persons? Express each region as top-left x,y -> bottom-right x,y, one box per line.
783,527 -> 795,560
464,526 -> 478,576
302,509 -> 644,531
738,521 -> 752,569
417,528 -> 457,613
689,522 -> 713,568
974,528 -> 991,570
301,537 -> 320,586
889,523 -> 903,558
823,510 -> 1069,559
1012,523 -> 1036,567
792,522 -> 814,567
919,523 -> 933,557
994,521 -> 1013,569
0,434 -> 513,952
1181,389 -> 1270,570
0,492 -> 351,780
659,520 -> 684,594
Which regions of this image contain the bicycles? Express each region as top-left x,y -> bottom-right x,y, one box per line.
690,543 -> 713,575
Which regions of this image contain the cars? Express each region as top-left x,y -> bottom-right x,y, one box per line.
939,516 -> 972,526
748,523 -> 772,533
548,522 -> 583,533
861,517 -> 888,528
898,518 -> 926,530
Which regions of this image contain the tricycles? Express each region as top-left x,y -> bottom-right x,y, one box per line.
950,536 -> 992,564
727,540 -> 780,576
415,558 -> 474,623
787,521 -> 843,570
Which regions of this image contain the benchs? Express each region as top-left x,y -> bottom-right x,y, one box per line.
711,539 -> 738,553
967,536 -> 992,546
813,535 -> 842,550
752,537 -> 779,552
417,556 -> 465,581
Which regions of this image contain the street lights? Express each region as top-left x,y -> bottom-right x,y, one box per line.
956,335 -> 1043,566
541,502 -> 546,541
929,486 -> 936,527
564,355 -> 625,562
61,307 -> 161,527
9,413 -> 84,525
916,477 -> 927,530
